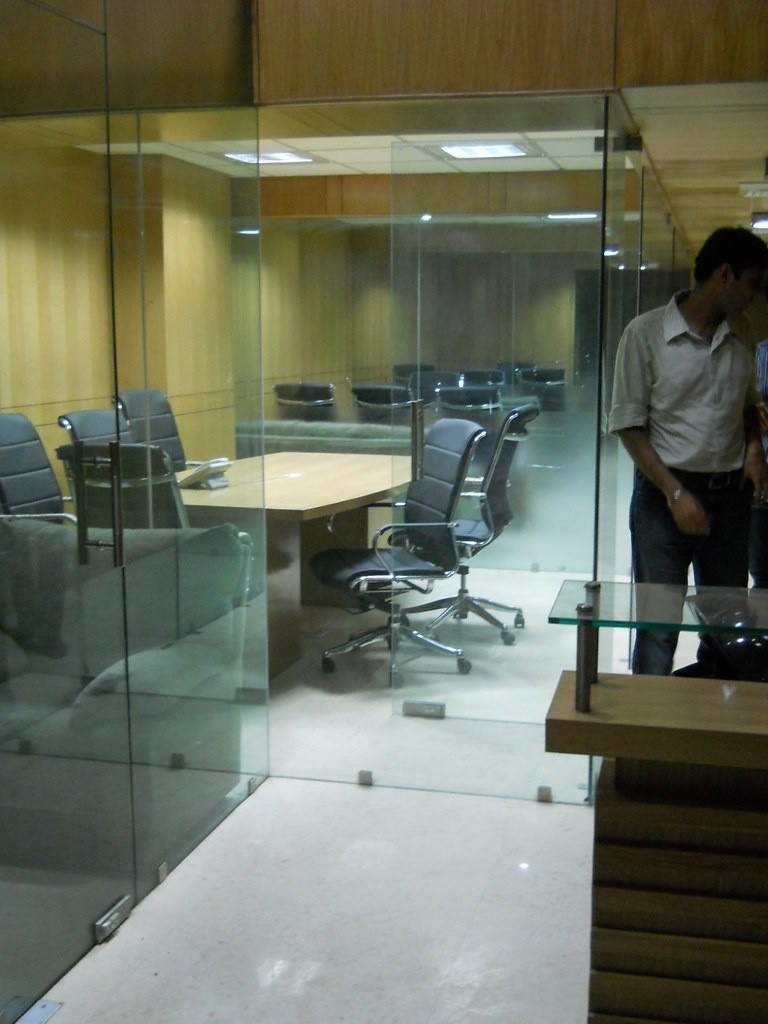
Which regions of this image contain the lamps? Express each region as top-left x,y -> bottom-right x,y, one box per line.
751,211 -> 768,229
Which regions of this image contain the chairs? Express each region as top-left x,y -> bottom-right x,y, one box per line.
58,409 -> 135,444
435,386 -> 508,486
351,385 -> 415,427
0,414 -> 78,526
309,418 -> 488,691
393,361 -> 567,412
387,404 -> 541,648
272,383 -> 334,421
111,391 -> 203,472
56,444 -> 189,528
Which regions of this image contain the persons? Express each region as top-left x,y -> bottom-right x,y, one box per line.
606,223 -> 768,676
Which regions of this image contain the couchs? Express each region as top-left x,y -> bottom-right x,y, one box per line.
235,421 -> 430,459
1,518 -> 255,886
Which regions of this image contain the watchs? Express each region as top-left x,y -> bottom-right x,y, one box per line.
666,486 -> 687,508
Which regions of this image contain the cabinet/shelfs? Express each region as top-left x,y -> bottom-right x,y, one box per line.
544,578 -> 768,1024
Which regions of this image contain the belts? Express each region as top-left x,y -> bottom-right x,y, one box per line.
668,469 -> 744,490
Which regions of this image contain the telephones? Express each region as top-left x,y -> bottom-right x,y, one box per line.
177,457 -> 234,489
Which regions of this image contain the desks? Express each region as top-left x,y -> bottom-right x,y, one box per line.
176,451 -> 412,679
